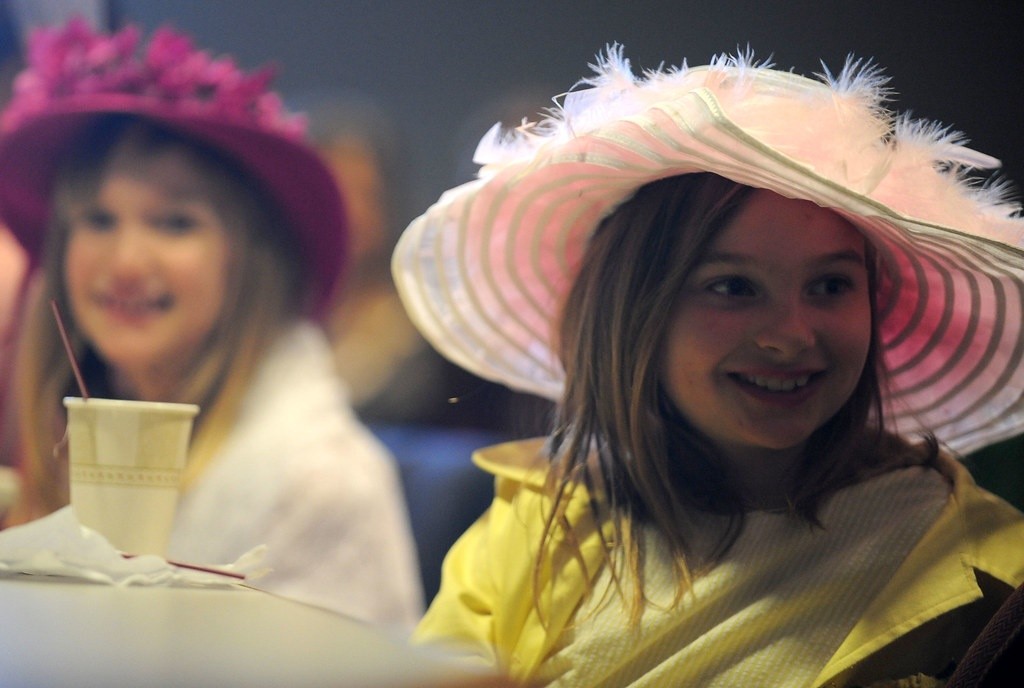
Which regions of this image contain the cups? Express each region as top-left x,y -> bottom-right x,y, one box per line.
62,397 -> 199,557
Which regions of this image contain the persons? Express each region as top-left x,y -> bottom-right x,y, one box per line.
390,43 -> 1024,688
0,14 -> 425,640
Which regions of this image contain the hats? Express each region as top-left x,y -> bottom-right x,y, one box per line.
391,45 -> 1024,457
2,22 -> 350,322
